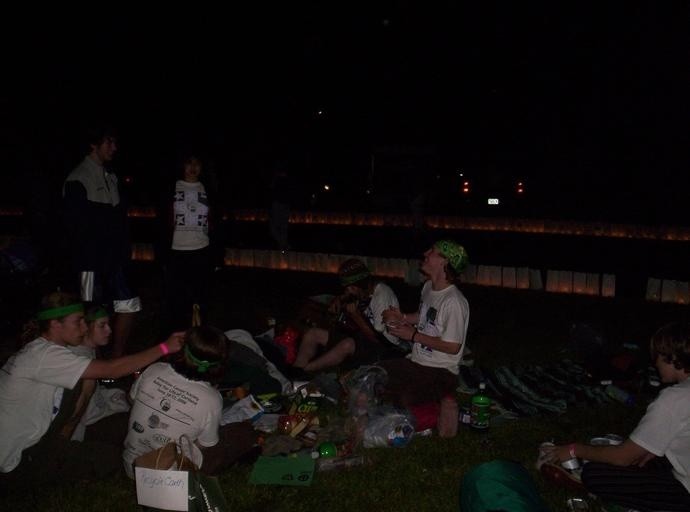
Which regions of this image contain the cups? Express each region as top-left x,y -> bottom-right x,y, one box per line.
538,442 -> 555,464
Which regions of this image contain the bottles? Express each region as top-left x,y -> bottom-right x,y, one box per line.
605,385 -> 635,406
437,393 -> 459,438
190,470 -> 225,512
310,440 -> 354,458
316,455 -> 365,471
470,382 -> 491,433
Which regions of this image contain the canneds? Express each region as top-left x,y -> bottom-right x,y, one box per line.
562,433 -> 624,481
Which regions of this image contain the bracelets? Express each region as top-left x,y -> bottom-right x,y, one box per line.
410,330 -> 419,340
158,342 -> 170,357
569,442 -> 578,459
403,313 -> 407,319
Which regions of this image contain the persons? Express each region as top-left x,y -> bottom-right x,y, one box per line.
48,306 -> 129,443
292,257 -> 399,375
532,318 -> 690,512
115,321 -> 257,490
62,131 -> 141,363
364,236 -> 474,431
156,148 -> 221,318
0,292 -> 193,476
